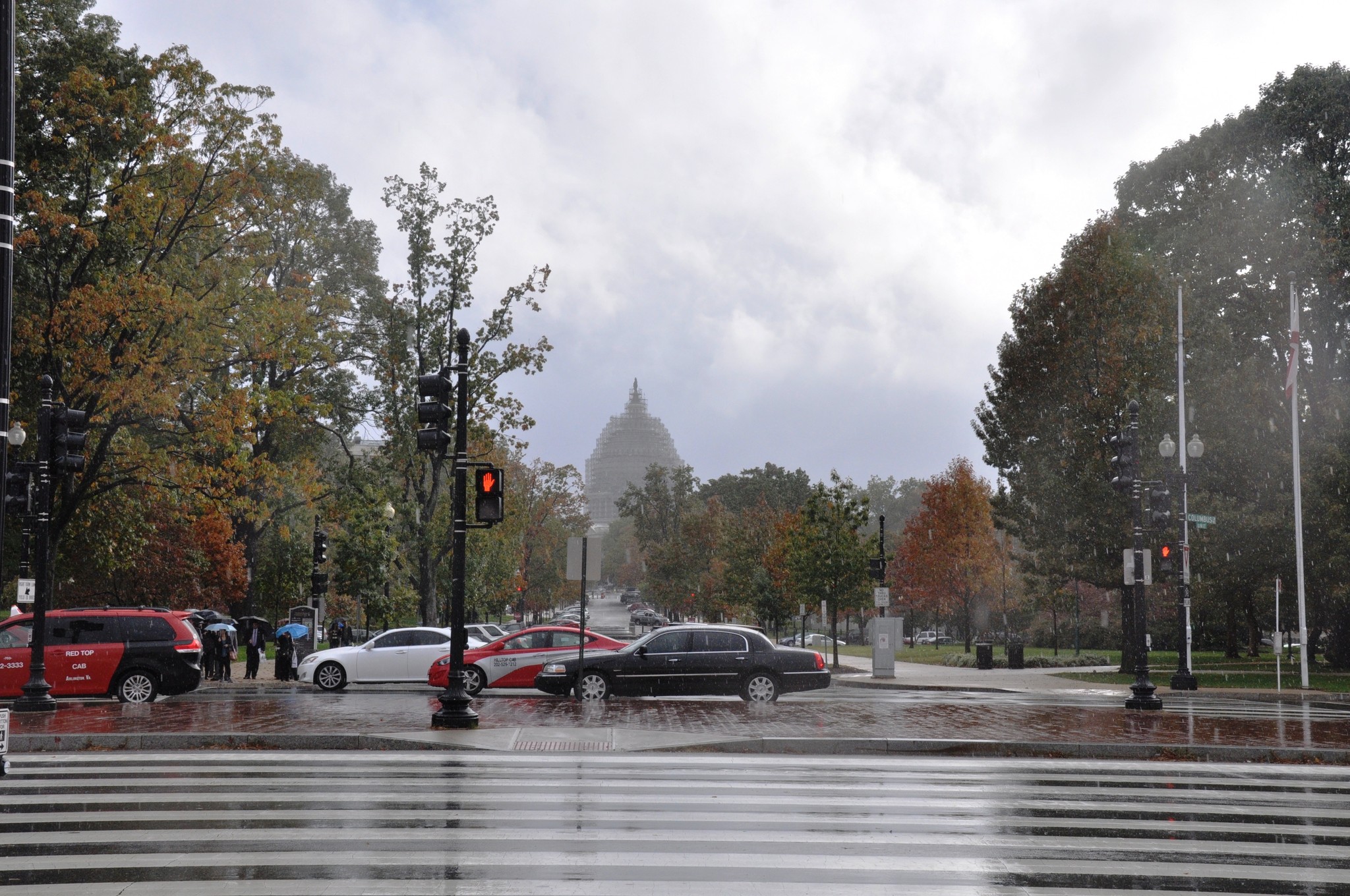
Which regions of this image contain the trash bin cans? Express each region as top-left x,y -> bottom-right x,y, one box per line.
1007,641 -> 1025,669
274,643 -> 282,680
975,639 -> 994,669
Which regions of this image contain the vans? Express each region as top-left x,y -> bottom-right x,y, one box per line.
0,605 -> 205,702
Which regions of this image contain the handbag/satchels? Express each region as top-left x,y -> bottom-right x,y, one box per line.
257,648 -> 267,664
229,645 -> 238,661
291,646 -> 298,668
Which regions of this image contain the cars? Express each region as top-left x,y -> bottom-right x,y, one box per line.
534,625 -> 831,701
296,627 -> 488,691
903,631 -> 1022,646
428,627 -> 630,696
621,593 -> 670,626
780,632 -> 846,648
444,603 -> 590,642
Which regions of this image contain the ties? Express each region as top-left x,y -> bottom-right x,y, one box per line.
252,630 -> 257,647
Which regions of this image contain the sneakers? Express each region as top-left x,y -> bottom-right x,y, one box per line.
252,677 -> 256,679
243,676 -> 250,679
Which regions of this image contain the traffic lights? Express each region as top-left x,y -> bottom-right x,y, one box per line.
474,470 -> 506,523
1158,541 -> 1174,573
1109,429 -> 1133,489
416,368 -> 453,457
312,572 -> 328,595
316,531 -> 327,564
1144,486 -> 1171,529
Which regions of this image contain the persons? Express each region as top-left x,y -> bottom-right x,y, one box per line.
339,621 -> 352,647
278,632 -> 294,682
242,622 -> 265,679
187,619 -> 238,683
327,623 -> 340,649
511,633 -> 543,649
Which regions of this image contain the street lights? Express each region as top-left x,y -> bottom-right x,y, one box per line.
1158,422 -> 1206,690
9,421 -> 38,614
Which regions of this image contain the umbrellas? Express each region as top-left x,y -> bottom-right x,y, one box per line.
329,617 -> 349,631
185,609 -> 238,639
275,623 -> 309,646
239,616 -> 274,645
